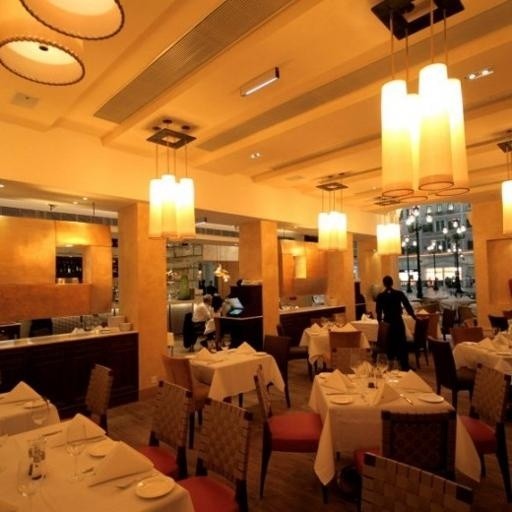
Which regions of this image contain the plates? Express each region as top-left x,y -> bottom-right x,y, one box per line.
255,352 -> 267,355
87,438 -> 120,457
328,394 -> 355,403
134,475 -> 176,499
416,392 -> 444,402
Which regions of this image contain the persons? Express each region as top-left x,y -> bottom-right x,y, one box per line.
192,295 -> 216,348
377,275 -> 420,373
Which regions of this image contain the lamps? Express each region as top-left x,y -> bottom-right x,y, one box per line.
370,195 -> 404,256
141,125 -> 203,242
215,262 -> 231,288
497,139 -> 512,237
370,0 -> 477,202
292,239 -> 314,282
314,179 -> 349,255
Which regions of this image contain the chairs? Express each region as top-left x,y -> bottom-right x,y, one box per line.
334,345 -> 374,375
329,312 -> 347,323
453,364 -> 512,502
486,312 -> 510,333
128,375 -> 192,485
352,405 -> 456,482
503,307 -> 512,323
445,326 -> 488,350
65,358 -> 112,434
260,330 -> 289,359
276,323 -> 312,379
163,396 -> 255,512
370,320 -> 395,360
456,304 -> 473,323
250,361 -> 331,501
327,327 -> 362,350
415,300 -> 439,312
264,343 -> 292,410
160,352 -> 216,450
420,307 -> 439,338
428,337 -> 475,413
402,313 -> 431,370
440,309 -> 456,342
354,450 -> 474,512
183,312 -> 210,352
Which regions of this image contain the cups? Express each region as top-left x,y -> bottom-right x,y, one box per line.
220,334 -> 231,352
63,422 -> 87,481
23,399 -> 50,446
376,352 -> 389,380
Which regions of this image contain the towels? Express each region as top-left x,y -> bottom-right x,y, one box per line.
310,323 -> 322,332
332,369 -> 356,388
0,381 -> 41,404
372,383 -> 401,405
493,335 -> 508,345
342,322 -> 357,331
321,373 -> 349,395
71,328 -> 84,336
66,413 -> 106,442
357,361 -> 374,375
235,341 -> 256,355
191,348 -> 217,361
361,314 -> 370,321
418,309 -> 429,314
395,369 -> 433,392
477,337 -> 496,351
86,441 -> 154,486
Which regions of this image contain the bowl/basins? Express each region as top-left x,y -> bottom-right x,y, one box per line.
119,323 -> 133,330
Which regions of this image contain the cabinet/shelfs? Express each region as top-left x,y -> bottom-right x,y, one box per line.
279,303 -> 346,358
0,324 -> 139,417
